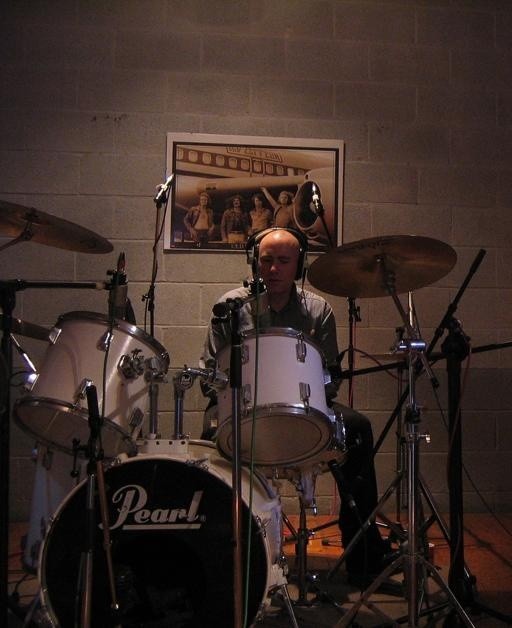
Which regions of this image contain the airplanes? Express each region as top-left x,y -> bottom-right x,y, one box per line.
170,143 -> 337,250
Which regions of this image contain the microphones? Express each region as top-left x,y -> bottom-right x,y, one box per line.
107,251 -> 131,323
211,287 -> 272,320
311,183 -> 322,211
243,279 -> 251,288
154,171 -> 179,204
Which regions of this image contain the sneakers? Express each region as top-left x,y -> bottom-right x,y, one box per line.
349,547 -> 404,587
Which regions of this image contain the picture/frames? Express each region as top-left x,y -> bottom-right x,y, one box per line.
162,131 -> 345,252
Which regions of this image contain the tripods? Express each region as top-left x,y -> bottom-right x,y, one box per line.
321,340 -> 479,628
323,403 -> 478,591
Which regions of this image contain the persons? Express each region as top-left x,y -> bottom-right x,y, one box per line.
184,193 -> 215,241
260,187 -> 298,230
220,195 -> 249,241
245,194 -> 273,239
199,226 -> 407,582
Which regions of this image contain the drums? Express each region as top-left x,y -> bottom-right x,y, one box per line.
38,438 -> 287,628
13,310 -> 170,458
213,326 -> 346,468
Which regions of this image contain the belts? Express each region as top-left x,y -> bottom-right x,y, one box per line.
229,232 -> 245,234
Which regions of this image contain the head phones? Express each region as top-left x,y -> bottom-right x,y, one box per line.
245,225 -> 309,281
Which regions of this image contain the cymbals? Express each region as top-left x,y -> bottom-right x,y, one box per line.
360,353 -> 440,361
308,234 -> 457,298
1,199 -> 113,254
